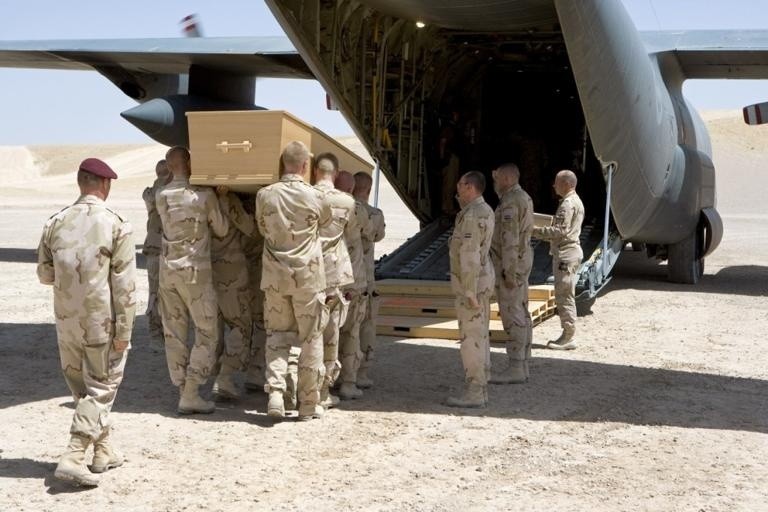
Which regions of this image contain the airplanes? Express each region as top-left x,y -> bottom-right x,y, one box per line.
0,0 -> 768,344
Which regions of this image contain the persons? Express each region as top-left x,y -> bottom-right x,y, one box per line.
444,171 -> 495,407
334,170 -> 374,399
282,152 -> 354,410
255,141 -> 334,420
489,163 -> 532,384
334,172 -> 385,387
531,170 -> 585,350
36,157 -> 137,487
140,146 -> 264,415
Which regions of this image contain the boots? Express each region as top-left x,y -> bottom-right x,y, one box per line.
488,358 -> 526,384
176,350 -> 374,421
493,360 -> 530,383
54,434 -> 100,486
446,383 -> 486,409
90,429 -> 125,473
547,331 -> 578,350
147,334 -> 165,356
449,382 -> 488,404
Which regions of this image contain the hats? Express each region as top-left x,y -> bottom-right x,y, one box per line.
80,158 -> 117,180
